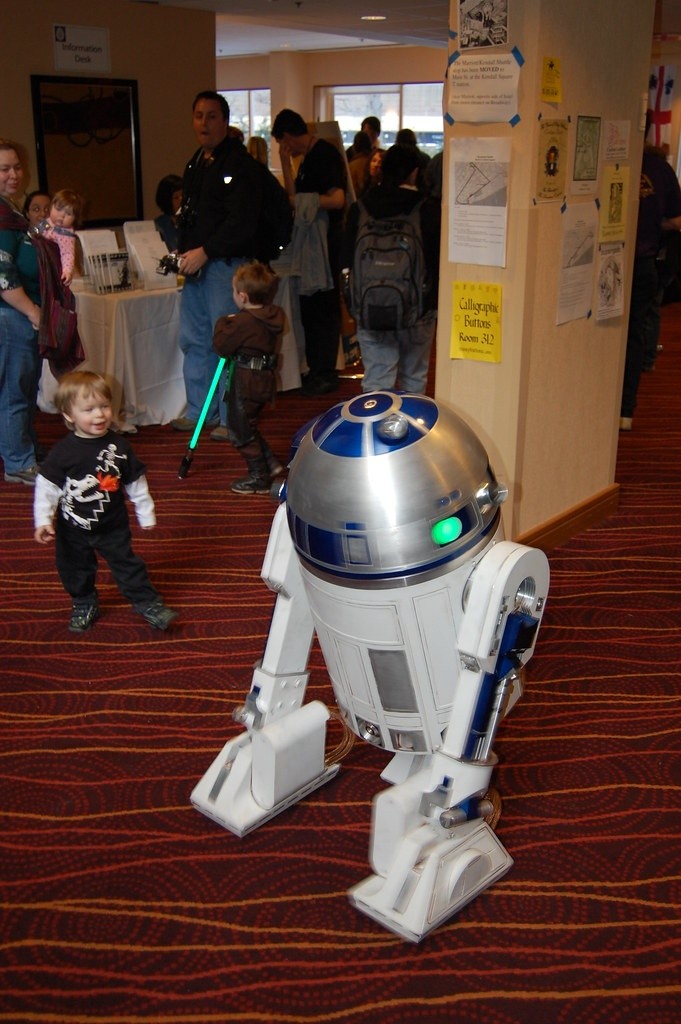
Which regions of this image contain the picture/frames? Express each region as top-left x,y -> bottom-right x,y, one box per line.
29,75 -> 144,227
51,23 -> 112,74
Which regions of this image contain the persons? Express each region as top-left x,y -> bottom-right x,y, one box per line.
20,189 -> 52,229
213,261 -> 286,495
37,189 -> 81,285
32,369 -> 176,635
152,85 -> 446,440
621,108 -> 681,435
0,142 -> 42,488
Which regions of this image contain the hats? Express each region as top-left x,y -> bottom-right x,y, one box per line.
396,129 -> 417,144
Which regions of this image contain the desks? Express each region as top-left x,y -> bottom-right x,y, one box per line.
35,268 -> 310,431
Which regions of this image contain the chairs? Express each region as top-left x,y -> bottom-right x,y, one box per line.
71,220 -> 158,280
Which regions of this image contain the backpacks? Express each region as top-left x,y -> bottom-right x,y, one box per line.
242,146 -> 294,262
348,198 -> 430,331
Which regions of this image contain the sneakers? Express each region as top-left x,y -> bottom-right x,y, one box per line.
231,471 -> 271,493
4,464 -> 41,486
68,602 -> 98,631
267,456 -> 282,477
132,595 -> 178,630
